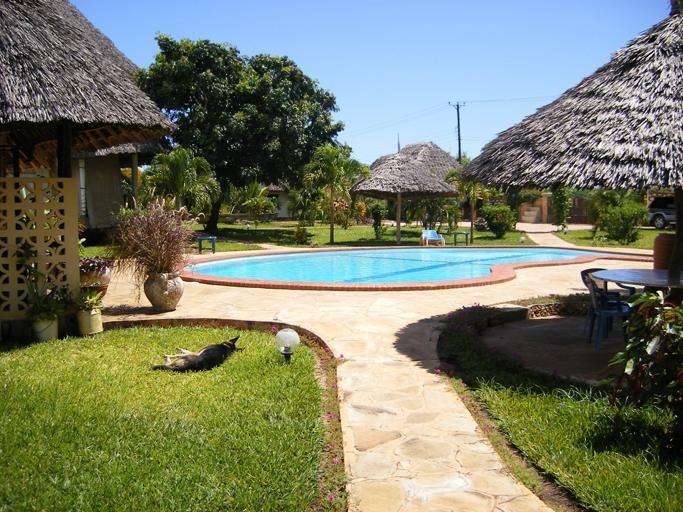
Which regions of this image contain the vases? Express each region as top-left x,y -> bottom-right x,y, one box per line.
145,270 -> 185,311
80,267 -> 111,299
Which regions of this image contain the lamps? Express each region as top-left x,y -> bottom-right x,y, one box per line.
274,327 -> 301,364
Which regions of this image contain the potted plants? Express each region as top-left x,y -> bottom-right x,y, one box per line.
27,282 -> 105,342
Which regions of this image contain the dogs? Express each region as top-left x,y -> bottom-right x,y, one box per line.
152,334 -> 246,373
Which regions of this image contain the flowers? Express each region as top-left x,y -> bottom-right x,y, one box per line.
78,254 -> 116,274
100,182 -> 206,306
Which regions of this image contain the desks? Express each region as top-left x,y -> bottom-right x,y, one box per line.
450,232 -> 470,246
592,269 -> 683,296
196,236 -> 217,254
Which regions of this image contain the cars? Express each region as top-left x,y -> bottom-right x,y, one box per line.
647,196 -> 677,230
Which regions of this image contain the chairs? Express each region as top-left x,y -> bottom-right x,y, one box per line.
419,229 -> 445,246
580,267 -> 635,345
